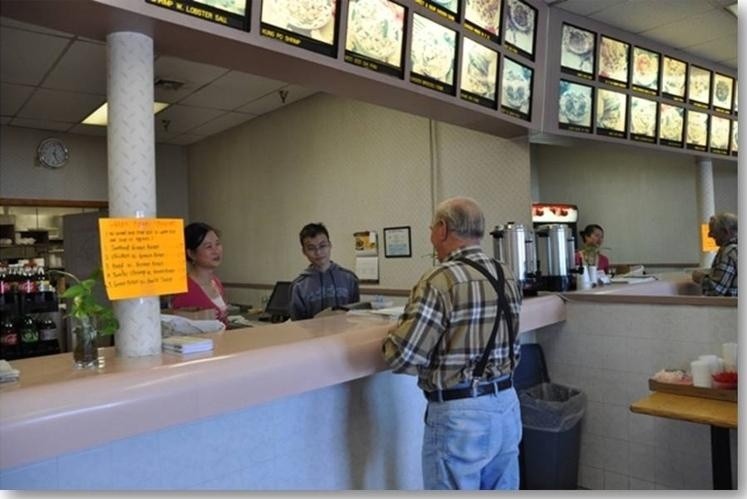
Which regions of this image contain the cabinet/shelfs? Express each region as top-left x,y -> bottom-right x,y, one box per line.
0,239 -> 69,361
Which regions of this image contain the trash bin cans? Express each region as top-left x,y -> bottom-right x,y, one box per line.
514,343 -> 587,489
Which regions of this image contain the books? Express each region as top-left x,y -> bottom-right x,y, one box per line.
162,335 -> 214,354
610,275 -> 658,284
0,359 -> 21,384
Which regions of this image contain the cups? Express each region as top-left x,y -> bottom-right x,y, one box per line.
691,343 -> 738,388
73,315 -> 98,369
576,266 -> 597,290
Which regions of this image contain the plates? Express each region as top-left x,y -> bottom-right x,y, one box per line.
282,1 -> 659,135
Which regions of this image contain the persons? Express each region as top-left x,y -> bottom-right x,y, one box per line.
168,222 -> 231,330
692,213 -> 738,296
289,221 -> 360,320
576,224 -> 609,275
382,196 -> 523,490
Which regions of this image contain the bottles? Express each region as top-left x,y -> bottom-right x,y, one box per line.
0,267 -> 59,355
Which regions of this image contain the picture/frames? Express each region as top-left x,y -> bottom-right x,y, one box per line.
384,227 -> 411,257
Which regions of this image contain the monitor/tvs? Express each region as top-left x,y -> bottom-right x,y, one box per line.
265,281 -> 293,323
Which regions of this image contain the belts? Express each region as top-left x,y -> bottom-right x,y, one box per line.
424,376 -> 512,403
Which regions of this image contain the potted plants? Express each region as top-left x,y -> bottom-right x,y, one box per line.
57,267 -> 119,369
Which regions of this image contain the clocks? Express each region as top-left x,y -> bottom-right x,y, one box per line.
38,138 -> 69,169
